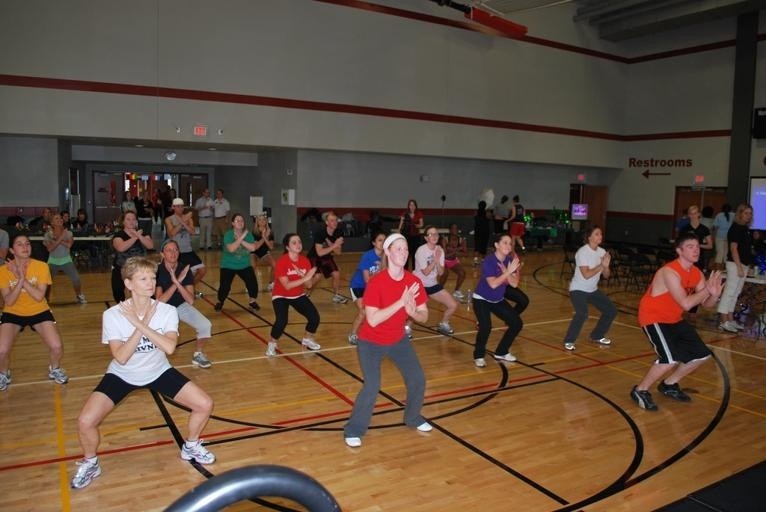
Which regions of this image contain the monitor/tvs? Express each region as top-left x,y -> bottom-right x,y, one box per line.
568,201 -> 590,222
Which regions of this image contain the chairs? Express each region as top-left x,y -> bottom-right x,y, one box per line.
625,254 -> 651,292
561,246 -> 579,278
620,249 -> 637,269
598,255 -> 619,288
638,247 -> 659,272
606,248 -> 618,271
612,249 -> 636,289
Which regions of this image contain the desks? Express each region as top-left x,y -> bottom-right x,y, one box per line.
27,235 -> 113,266
390,228 -> 460,245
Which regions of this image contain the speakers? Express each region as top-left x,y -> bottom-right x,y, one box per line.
753,107 -> 766,139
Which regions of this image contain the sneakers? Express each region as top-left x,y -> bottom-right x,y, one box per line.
48,367 -> 69,384
70,457 -> 102,490
475,356 -> 487,368
181,442 -> 217,465
493,350 -> 516,362
0,369 -> 12,390
265,342 -> 276,355
268,282 -> 274,291
191,351 -> 211,368
406,323 -> 414,339
75,294 -> 87,304
332,294 -> 348,305
249,300 -> 261,310
302,338 -> 320,349
348,335 -> 359,344
589,336 -> 611,345
657,381 -> 693,402
719,324 -> 736,332
417,420 -> 433,433
195,290 -> 204,298
453,289 -> 464,298
630,387 -> 658,411
213,301 -> 225,312
440,322 -> 455,334
730,321 -> 744,331
346,437 -> 364,447
564,341 -> 576,350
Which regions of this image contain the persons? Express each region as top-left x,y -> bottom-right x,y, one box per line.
398,199 -> 423,272
678,205 -> 712,313
196,188 -> 215,250
630,231 -> 725,411
29,208 -> 52,263
700,205 -> 715,272
214,189 -> 231,249
152,185 -> 177,239
112,210 -> 154,303
266,233 -> 321,356
73,208 -> 89,274
714,202 -> 736,270
0,232 -> 69,391
493,195 -> 511,244
305,212 -> 349,304
564,225 -> 617,351
155,240 -> 212,368
135,190 -> 153,255
471,232 -> 523,367
343,234 -> 432,448
250,211 -> 277,293
214,213 -> 260,312
0,229 -> 9,265
44,214 -> 87,306
348,230 -> 387,345
60,210 -> 73,230
474,201 -> 490,262
71,256 -> 214,489
507,195 -> 527,254
716,204 -> 753,335
404,226 -> 458,340
163,198 -> 206,299
122,191 -> 137,214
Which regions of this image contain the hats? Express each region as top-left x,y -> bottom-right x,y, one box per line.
172,198 -> 184,207
